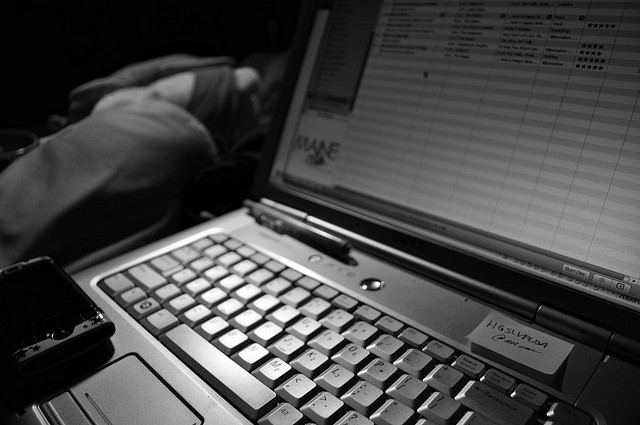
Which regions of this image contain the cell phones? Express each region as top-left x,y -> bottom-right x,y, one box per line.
1,257 -> 116,368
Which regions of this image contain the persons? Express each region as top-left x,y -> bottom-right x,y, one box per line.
0,42 -> 289,267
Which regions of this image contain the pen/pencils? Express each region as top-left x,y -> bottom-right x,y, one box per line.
242,199 -> 351,256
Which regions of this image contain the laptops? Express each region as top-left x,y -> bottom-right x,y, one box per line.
3,0 -> 636,425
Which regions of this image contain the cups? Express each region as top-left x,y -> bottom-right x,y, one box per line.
0,128 -> 42,172
